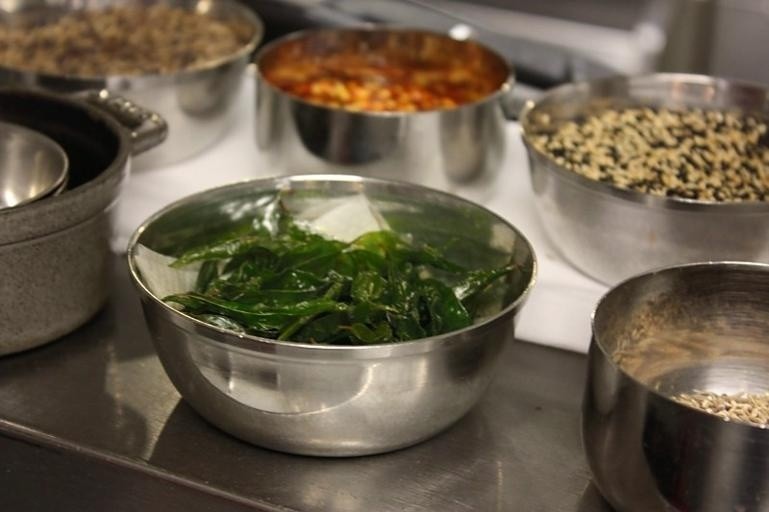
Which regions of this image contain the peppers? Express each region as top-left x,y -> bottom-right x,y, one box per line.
159,211 -> 514,346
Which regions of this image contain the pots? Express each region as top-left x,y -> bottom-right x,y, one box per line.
0,72 -> 169,364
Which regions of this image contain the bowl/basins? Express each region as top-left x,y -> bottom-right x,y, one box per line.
585,261 -> 769,512
519,72 -> 769,285
254,29 -> 517,202
0,0 -> 264,171
0,119 -> 71,213
124,171 -> 538,459
